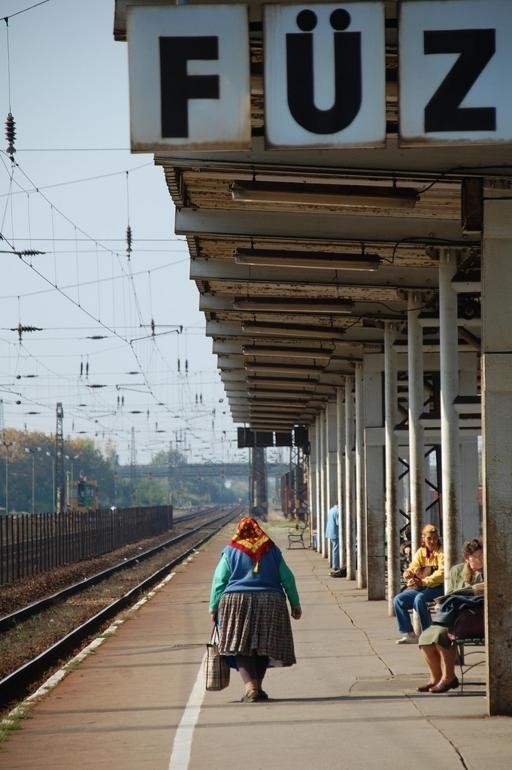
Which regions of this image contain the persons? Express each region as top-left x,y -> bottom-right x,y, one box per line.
208,514 -> 303,702
392,523 -> 445,645
325,502 -> 341,574
416,536 -> 486,694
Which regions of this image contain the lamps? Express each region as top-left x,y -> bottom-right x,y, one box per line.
233,238 -> 382,272
229,171 -> 419,209
240,323 -> 347,434
233,283 -> 356,314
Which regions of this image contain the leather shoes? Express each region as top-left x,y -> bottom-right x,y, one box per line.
417,676 -> 459,693
240,689 -> 269,703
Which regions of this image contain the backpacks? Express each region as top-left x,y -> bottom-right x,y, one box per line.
329,566 -> 347,578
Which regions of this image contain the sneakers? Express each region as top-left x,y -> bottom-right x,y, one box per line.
396,633 -> 418,644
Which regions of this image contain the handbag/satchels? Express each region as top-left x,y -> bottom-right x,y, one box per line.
205,619 -> 230,692
411,565 -> 435,590
447,605 -> 485,640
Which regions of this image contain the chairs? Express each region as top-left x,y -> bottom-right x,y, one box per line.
288,521 -> 307,549
449,638 -> 487,694
399,584 -> 444,613
